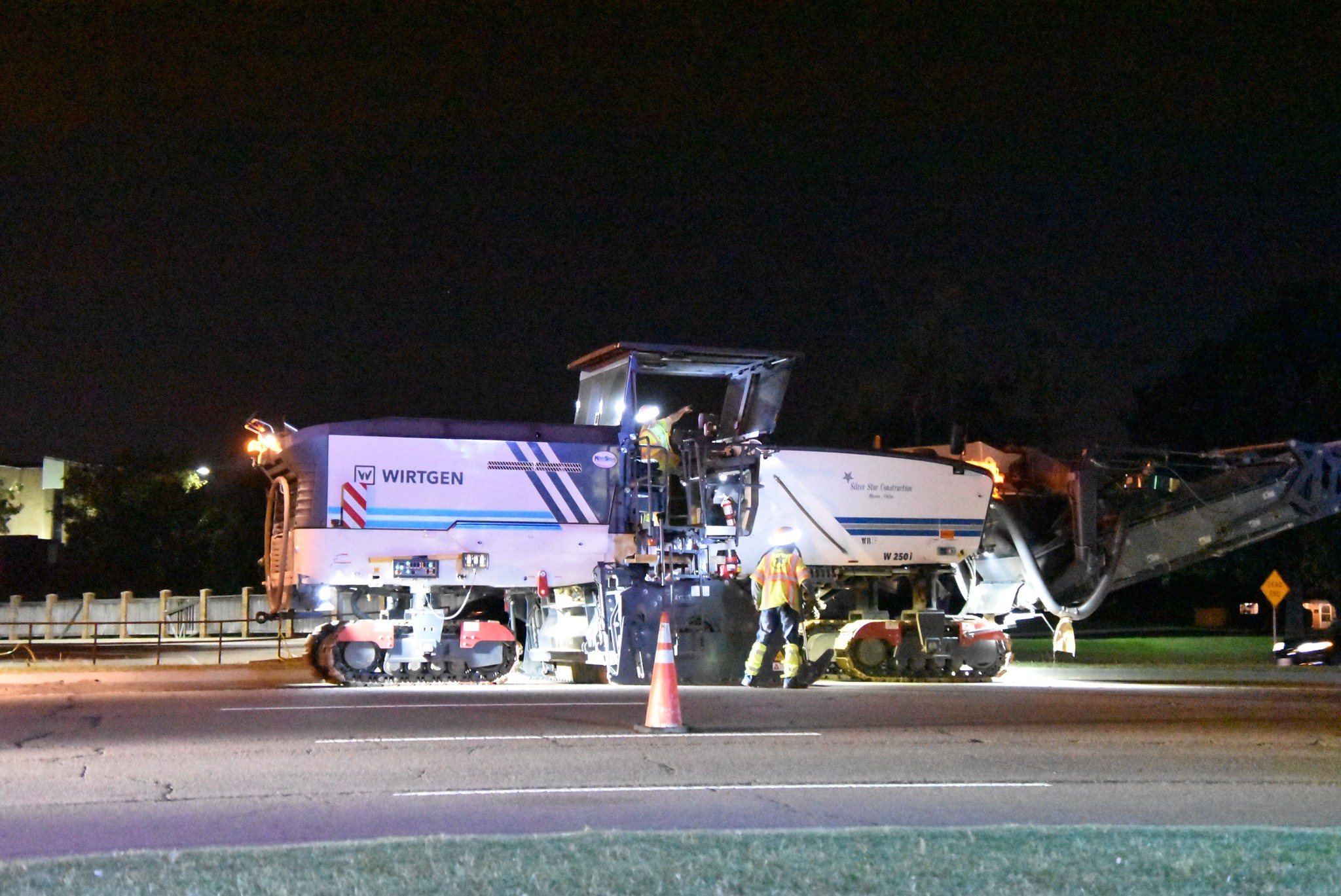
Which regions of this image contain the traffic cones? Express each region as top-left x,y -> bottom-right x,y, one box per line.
635,611 -> 689,739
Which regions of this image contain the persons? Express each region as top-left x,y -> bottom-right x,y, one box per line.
741,526 -> 816,689
634,403 -> 693,527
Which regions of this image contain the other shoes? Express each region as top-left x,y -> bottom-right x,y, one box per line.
783,678 -> 809,688
742,675 -> 760,686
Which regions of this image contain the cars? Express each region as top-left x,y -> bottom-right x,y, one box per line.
1272,614 -> 1341,666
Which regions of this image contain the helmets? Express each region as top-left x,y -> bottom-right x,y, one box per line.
636,405 -> 659,423
769,526 -> 798,546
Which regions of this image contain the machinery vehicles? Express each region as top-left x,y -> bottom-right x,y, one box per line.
255,342 -> 1340,684
